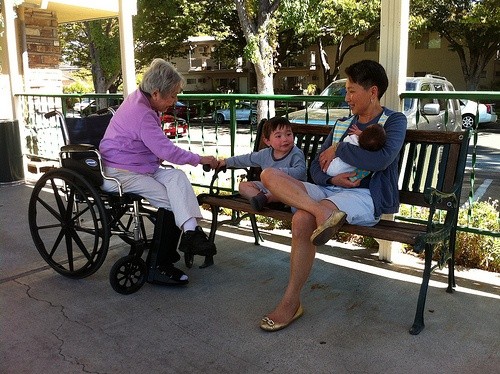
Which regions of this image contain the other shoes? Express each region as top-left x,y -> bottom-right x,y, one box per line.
249,192 -> 267,212
259,301 -> 303,331
310,209 -> 347,247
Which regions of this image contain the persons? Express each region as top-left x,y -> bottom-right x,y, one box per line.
218,117 -> 307,214
323,123 -> 387,183
98,56 -> 218,284
259,59 -> 408,332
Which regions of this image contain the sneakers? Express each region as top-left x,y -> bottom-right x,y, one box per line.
178,226 -> 218,257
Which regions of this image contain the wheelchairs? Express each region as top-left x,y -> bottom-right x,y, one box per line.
28,107 -> 194,295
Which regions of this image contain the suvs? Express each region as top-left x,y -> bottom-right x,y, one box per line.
281,73 -> 462,175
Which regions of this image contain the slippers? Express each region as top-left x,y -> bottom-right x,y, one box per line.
160,266 -> 189,282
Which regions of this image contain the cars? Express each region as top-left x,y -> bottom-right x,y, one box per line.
459,98 -> 498,129
73,99 -> 96,114
213,101 -> 258,125
158,102 -> 198,138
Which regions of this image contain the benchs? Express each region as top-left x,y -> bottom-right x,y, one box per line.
197,118 -> 473,335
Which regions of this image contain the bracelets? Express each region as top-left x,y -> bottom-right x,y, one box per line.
332,142 -> 337,154
